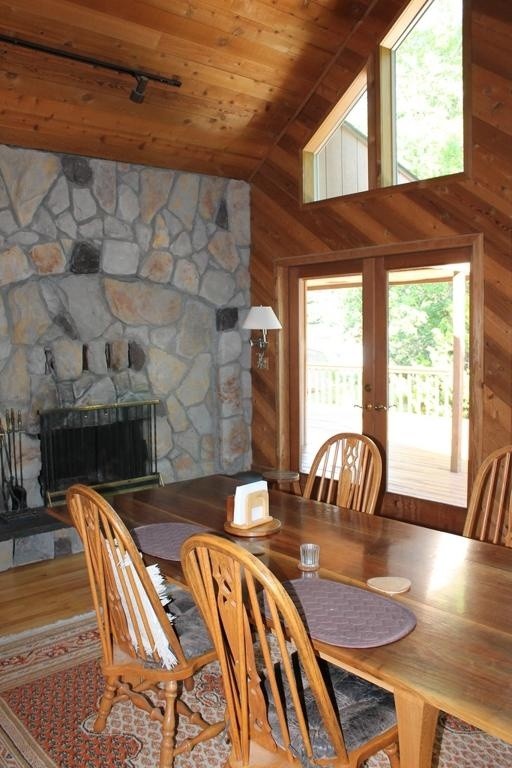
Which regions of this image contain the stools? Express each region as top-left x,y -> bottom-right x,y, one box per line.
262,471 -> 302,497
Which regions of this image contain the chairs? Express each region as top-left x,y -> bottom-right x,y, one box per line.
462,446 -> 512,549
65,484 -> 231,768
303,433 -> 383,515
181,534 -> 402,768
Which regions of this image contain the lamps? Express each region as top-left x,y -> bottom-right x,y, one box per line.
241,305 -> 283,348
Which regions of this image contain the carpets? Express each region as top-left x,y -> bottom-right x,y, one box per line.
0,606 -> 512,768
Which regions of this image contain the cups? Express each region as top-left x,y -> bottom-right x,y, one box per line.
299,543 -> 320,567
302,571 -> 320,580
